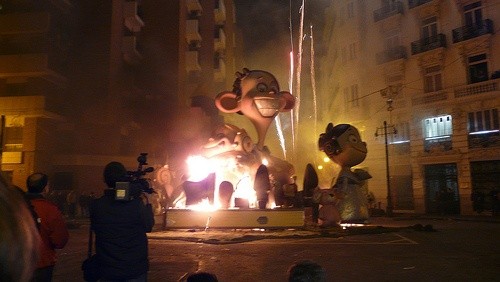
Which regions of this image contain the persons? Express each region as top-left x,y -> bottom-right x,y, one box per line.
0,161 -> 155,282
302,164 -> 320,230
473,186 -> 500,216
254,164 -> 272,210
317,123 -> 375,223
287,259 -> 326,282
155,67 -> 295,210
384,196 -> 393,216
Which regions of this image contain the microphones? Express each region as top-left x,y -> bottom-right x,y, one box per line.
144,167 -> 155,173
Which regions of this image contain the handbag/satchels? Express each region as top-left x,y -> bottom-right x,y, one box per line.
82,254 -> 101,282
